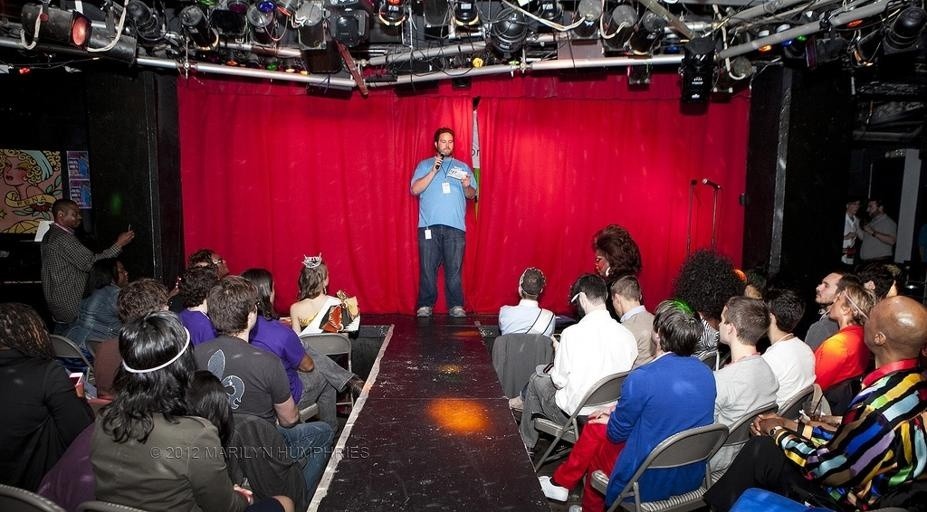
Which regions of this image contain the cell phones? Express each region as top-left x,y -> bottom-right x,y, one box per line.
552,334 -> 561,343
69,372 -> 83,386
128,224 -> 132,231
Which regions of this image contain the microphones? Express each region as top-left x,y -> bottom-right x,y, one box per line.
703,178 -> 719,190
435,152 -> 445,170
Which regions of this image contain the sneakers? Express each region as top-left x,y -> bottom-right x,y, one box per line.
417,307 -> 432,317
509,397 -> 523,411
448,305 -> 467,317
569,504 -> 582,512
538,476 -> 569,503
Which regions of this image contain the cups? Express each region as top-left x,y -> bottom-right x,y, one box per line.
801,401 -> 821,421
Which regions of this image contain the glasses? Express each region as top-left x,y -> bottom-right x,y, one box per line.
214,259 -> 222,263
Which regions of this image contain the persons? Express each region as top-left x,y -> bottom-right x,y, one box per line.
38,199 -> 134,338
498,223 -> 926,512
2,225 -> 366,511
843,199 -> 864,268
0,150 -> 61,235
408,127 -> 477,318
856,199 -> 897,264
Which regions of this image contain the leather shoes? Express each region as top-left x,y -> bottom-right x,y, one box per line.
346,376 -> 364,395
